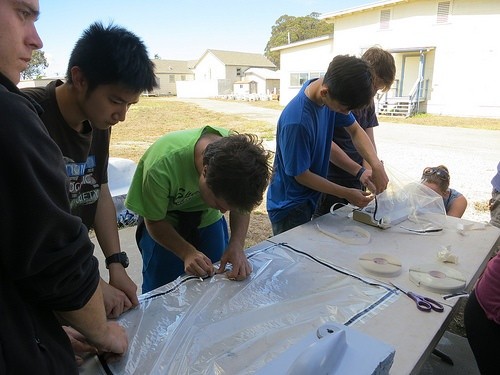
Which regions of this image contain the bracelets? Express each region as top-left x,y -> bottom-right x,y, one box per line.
355,167 -> 367,182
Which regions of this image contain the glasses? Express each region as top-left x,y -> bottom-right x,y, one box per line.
423,167 -> 448,181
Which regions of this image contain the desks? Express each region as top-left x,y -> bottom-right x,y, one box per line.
79,197 -> 500,375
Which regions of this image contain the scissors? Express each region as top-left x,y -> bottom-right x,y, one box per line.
390,282 -> 445,312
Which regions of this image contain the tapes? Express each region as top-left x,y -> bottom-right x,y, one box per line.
317,325 -> 340,340
409,261 -> 466,294
358,251 -> 403,278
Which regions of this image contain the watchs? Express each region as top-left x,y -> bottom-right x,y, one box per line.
105,251 -> 129,269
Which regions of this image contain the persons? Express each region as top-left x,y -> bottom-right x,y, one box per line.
123,124 -> 274,295
0,0 -> 128,375
420,165 -> 468,218
266,54 -> 377,237
20,21 -> 160,319
464,249 -> 500,375
311,47 -> 397,221
